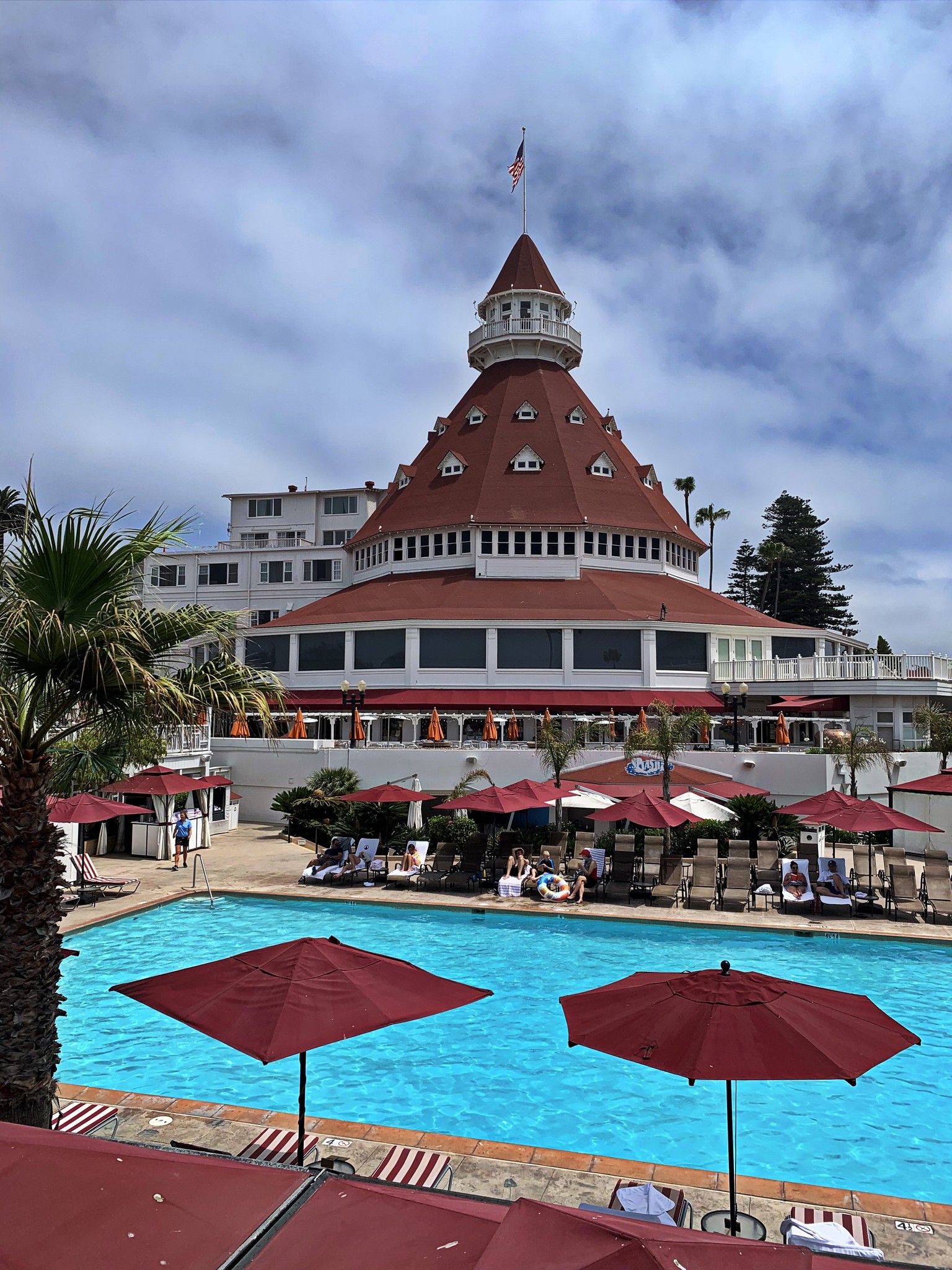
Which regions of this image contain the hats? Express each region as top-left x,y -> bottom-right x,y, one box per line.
579,849 -> 590,856
827,860 -> 836,866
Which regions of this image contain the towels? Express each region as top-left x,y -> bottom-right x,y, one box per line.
332,837 -> 352,853
371,859 -> 384,870
756,884 -> 772,894
470,871 -> 483,883
411,865 -> 422,871
582,847 -> 605,878
498,863 -> 532,897
817,857 -> 852,906
782,858 -> 815,901
855,891 -> 867,898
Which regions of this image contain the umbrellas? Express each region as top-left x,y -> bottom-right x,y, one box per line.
427,707 -> 444,748
608,708 -> 616,749
772,786 -> 864,858
558,961 -> 920,1237
338,778 -> 741,882
635,707 -> 649,749
482,707 -> 554,744
348,707 -> 365,748
196,707 -> 308,739
776,711 -> 790,752
700,710 -> 709,751
801,795 -> 945,903
108,937 -> 495,1166
45,792 -> 155,898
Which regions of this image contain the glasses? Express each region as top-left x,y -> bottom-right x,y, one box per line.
333,843 -> 340,847
793,867 -> 798,869
828,866 -> 836,868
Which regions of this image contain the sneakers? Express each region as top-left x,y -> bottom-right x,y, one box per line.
364,882 -> 369,885
365,882 -> 374,886
172,865 -> 179,870
311,866 -> 317,876
298,876 -> 306,882
183,863 -> 188,867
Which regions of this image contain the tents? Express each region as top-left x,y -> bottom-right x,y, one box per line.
91,765 -> 218,859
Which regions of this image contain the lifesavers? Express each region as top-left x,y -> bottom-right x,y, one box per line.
536,873 -> 570,903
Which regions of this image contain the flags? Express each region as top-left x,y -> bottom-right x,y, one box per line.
507,139 -> 524,193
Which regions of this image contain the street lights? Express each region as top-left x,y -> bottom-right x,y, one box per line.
721,682 -> 748,752
340,679 -> 367,748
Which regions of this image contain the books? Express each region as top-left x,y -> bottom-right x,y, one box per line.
790,873 -> 802,882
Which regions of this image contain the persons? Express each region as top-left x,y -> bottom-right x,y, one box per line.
816,860 -> 849,899
503,847 -> 597,903
298,838 -> 366,882
783,860 -> 808,900
400,844 -> 421,872
172,811 -> 192,870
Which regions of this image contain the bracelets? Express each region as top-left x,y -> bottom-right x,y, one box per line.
173,834 -> 175,835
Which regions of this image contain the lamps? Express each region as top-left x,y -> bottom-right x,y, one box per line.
896,759 -> 907,767
201,755 -> 212,762
743,760 -> 756,768
659,603 -> 668,621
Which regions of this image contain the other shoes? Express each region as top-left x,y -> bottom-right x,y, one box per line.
395,883 -> 405,888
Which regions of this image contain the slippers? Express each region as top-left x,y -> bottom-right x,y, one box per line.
565,899 -> 571,902
487,888 -> 491,892
852,914 -> 868,919
488,889 -> 495,894
567,900 -> 576,903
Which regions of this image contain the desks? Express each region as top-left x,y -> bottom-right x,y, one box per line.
370,867 -> 389,885
76,887 -> 100,908
672,861 -> 692,879
304,1160 -> 356,1175
854,893 -> 879,918
634,881 -> 654,903
700,1209 -> 767,1242
752,890 -> 776,911
557,877 -> 575,895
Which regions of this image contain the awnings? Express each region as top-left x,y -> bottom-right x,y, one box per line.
261,688 -> 737,713
766,695 -> 850,711
198,775 -> 233,788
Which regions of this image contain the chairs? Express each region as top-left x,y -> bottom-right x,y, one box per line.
601,744 -> 624,751
386,831 -> 952,925
780,1206 -> 885,1260
369,742 -> 423,749
371,1145 -> 454,1192
301,836 -> 381,886
58,852 -> 140,912
717,745 -> 752,753
462,740 -> 530,750
51,1100 -> 120,1138
579,1178 -> 693,1230
335,740 -> 351,748
170,1128 -> 320,1167
